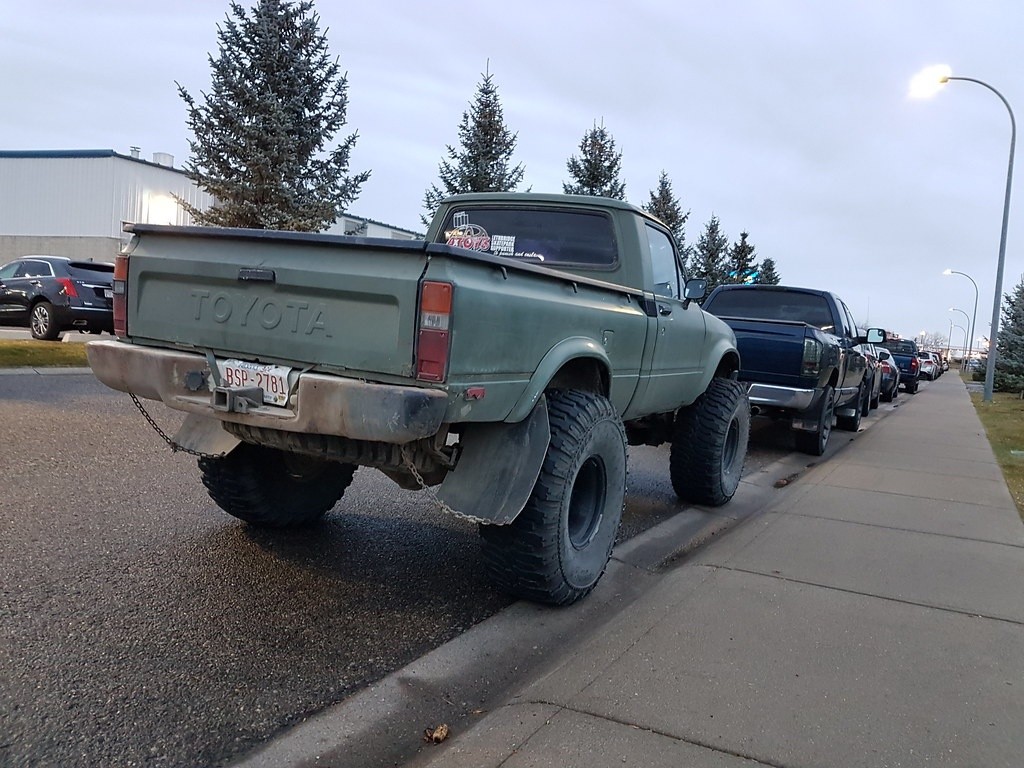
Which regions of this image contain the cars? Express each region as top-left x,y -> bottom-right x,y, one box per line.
875,346 -> 900,403
857,326 -> 891,417
965,359 -> 982,372
918,351 -> 950,381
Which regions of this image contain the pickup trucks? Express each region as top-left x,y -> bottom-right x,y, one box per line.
702,285 -> 887,456
882,339 -> 922,394
84,192 -> 752,608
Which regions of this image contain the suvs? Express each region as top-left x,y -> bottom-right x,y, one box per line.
0,254 -> 115,341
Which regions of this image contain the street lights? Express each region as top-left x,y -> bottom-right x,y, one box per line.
909,63 -> 1017,405
949,307 -> 969,372
952,325 -> 966,370
943,268 -> 979,372
